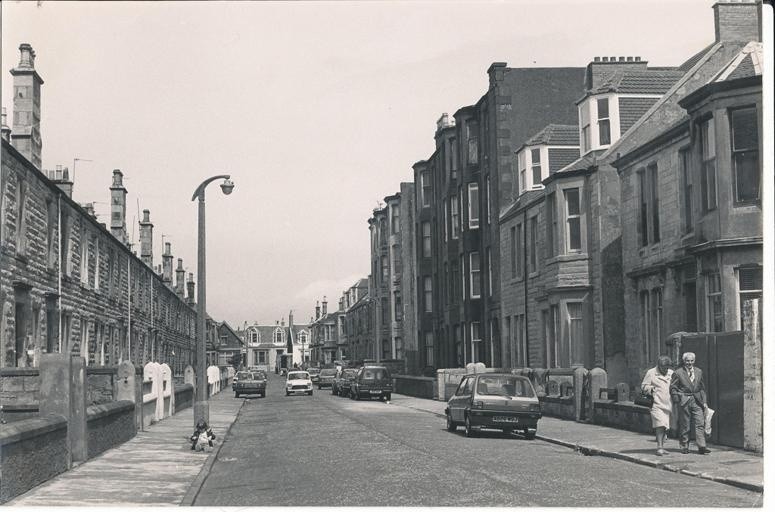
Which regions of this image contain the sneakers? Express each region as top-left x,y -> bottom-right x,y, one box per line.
656,448 -> 669,455
196,443 -> 213,452
681,447 -> 710,454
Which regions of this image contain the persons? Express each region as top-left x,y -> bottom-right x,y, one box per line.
669,351 -> 712,455
641,356 -> 676,456
190,419 -> 215,452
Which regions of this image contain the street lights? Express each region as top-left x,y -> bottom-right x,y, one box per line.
192,174 -> 236,437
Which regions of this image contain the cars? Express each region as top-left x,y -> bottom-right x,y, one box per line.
232,367 -> 268,397
279,358 -> 394,401
444,371 -> 541,440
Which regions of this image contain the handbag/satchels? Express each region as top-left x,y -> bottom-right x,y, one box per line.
704,408 -> 715,434
634,384 -> 653,408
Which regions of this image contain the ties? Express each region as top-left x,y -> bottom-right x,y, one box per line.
689,368 -> 695,385
